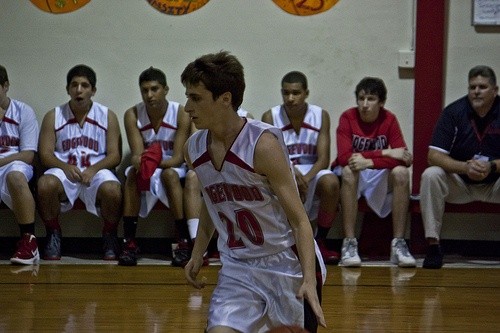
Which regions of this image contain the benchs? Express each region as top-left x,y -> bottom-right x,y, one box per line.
0,197 -> 375,213
409,199 -> 500,215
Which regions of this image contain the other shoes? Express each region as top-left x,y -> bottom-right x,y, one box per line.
316,241 -> 339,263
118,242 -> 140,266
423,244 -> 443,269
173,247 -> 190,266
202,251 -> 209,267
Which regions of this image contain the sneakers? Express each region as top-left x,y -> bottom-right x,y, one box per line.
10,235 -> 40,264
339,238 -> 361,266
102,236 -> 116,259
390,238 -> 416,266
43,230 -> 62,260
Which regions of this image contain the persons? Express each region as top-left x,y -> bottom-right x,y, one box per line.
419,66 -> 500,269
0,65 -> 40,265
117,66 -> 190,268
261,71 -> 341,265
181,49 -> 328,333
189,108 -> 255,265
331,76 -> 416,268
35,64 -> 123,261
